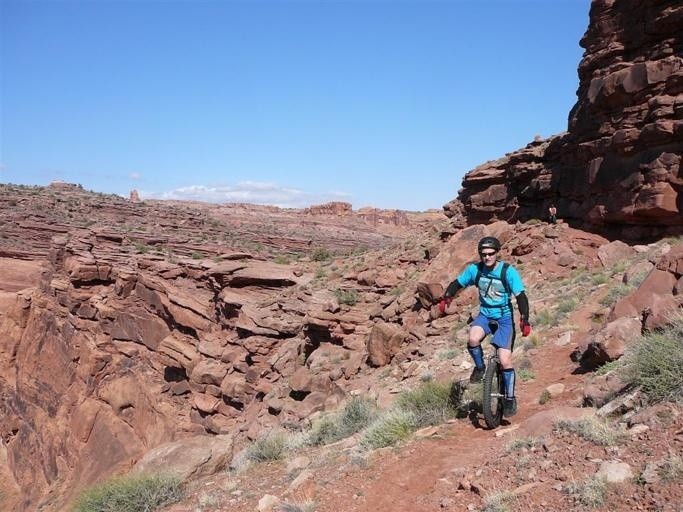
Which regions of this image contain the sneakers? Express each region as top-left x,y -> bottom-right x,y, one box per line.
470,364 -> 486,384
504,397 -> 517,418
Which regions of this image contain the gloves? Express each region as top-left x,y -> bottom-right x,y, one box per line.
520,319 -> 530,337
439,297 -> 451,314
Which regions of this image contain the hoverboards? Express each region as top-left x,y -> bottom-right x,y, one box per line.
470,321 -> 516,431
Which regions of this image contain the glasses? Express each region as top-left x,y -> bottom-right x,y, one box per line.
481,251 -> 497,257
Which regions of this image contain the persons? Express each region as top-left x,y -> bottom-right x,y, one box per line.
440,236 -> 530,418
548,204 -> 556,223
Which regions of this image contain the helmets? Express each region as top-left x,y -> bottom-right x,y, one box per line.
478,237 -> 500,251
